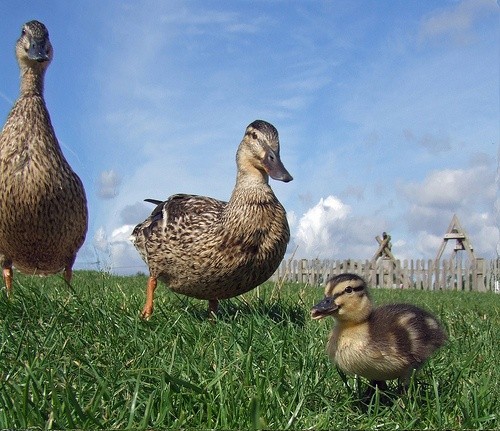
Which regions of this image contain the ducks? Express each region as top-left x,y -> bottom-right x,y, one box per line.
311,274 -> 448,411
0,21 -> 89,300
130,120 -> 293,321
